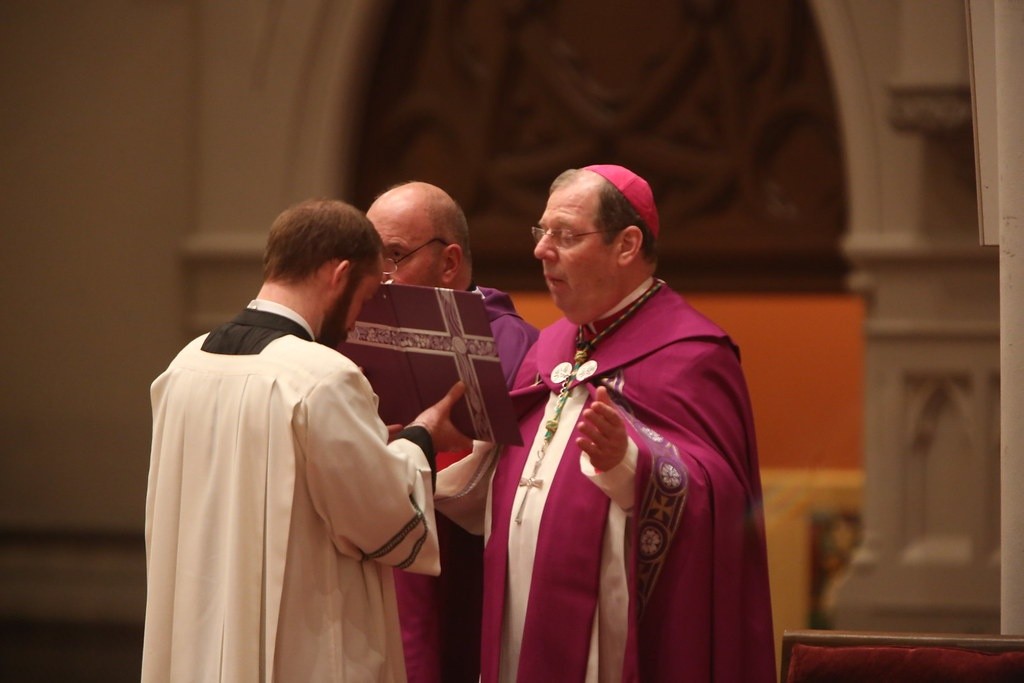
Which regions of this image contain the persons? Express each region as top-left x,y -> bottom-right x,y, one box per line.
362,180 -> 545,683
432,160 -> 778,683
136,197 -> 473,682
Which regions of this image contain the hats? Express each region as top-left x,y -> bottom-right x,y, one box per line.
584,164 -> 658,241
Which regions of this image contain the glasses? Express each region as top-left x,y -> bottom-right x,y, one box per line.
532,226 -> 633,247
381,237 -> 449,274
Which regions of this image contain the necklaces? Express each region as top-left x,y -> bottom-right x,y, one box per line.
544,281 -> 662,444
513,273 -> 665,527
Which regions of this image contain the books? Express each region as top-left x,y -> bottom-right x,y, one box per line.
325,279 -> 527,449
